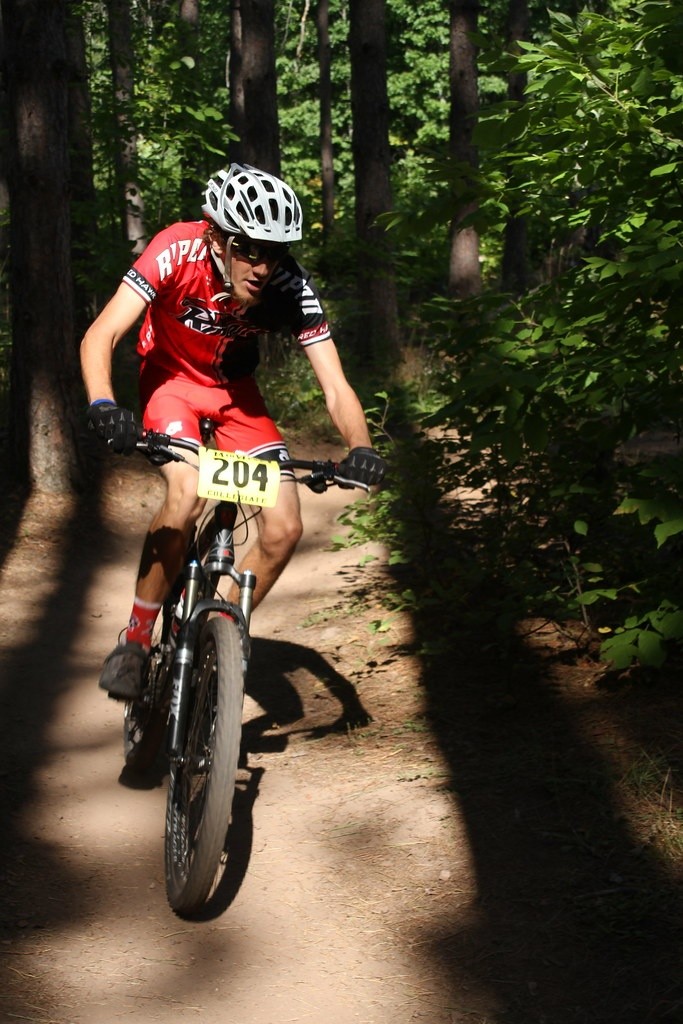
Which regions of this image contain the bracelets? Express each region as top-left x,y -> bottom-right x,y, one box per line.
92,400 -> 116,408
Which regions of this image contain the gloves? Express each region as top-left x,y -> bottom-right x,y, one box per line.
89,403 -> 137,457
336,447 -> 385,489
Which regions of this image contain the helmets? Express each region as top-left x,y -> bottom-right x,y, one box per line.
201,163 -> 304,243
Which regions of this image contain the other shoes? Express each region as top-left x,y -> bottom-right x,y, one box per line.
204,674 -> 218,724
99,642 -> 145,699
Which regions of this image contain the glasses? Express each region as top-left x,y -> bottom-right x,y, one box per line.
214,224 -> 289,265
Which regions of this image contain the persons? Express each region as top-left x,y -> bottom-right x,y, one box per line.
78,162 -> 394,698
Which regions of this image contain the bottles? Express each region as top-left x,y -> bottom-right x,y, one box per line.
168,586 -> 185,649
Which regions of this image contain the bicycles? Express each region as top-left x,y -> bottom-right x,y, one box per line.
86,416 -> 404,923
